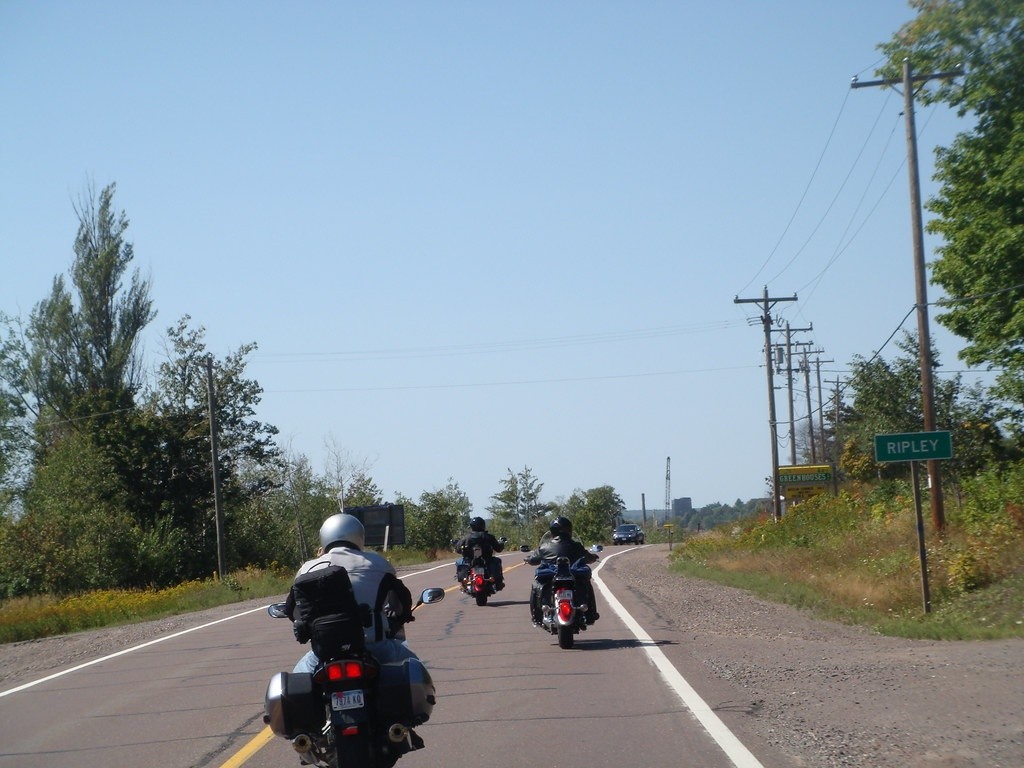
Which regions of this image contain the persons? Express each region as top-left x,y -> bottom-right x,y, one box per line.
524,517 -> 600,625
455,516 -> 507,592
285,513 -> 425,766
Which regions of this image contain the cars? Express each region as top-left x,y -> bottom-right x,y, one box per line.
612,524 -> 645,545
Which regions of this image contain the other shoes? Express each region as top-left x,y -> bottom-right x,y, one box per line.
496,582 -> 506,591
587,612 -> 600,626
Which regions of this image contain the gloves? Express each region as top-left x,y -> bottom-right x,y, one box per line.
396,611 -> 412,623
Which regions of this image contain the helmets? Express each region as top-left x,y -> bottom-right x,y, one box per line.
550,516 -> 573,540
470,517 -> 486,531
319,514 -> 365,550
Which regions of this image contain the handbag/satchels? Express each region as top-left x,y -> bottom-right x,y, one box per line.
289,566 -> 365,648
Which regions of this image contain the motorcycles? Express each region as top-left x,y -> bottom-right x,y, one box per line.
450,526 -> 508,606
264,587 -> 445,768
519,529 -> 603,650
